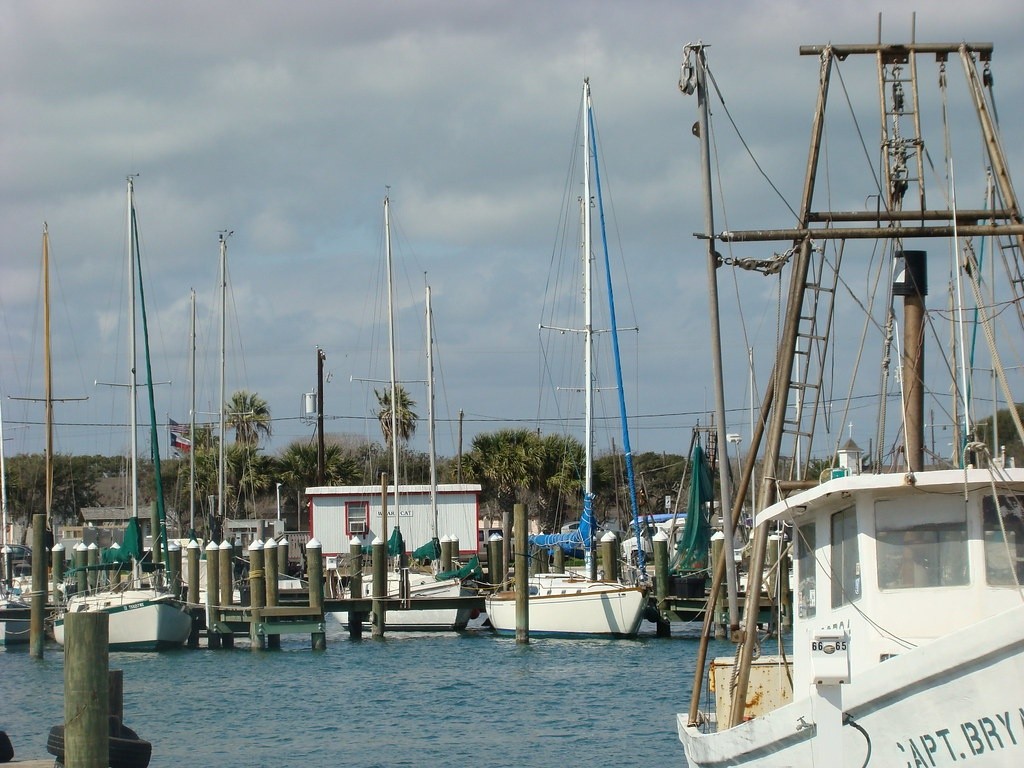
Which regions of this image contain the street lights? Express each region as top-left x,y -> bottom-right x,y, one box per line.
276,482 -> 283,521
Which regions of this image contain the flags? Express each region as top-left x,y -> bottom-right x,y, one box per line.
168,433 -> 192,451
169,420 -> 191,433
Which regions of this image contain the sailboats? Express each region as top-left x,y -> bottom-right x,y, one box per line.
0,0 -> 1023,768
